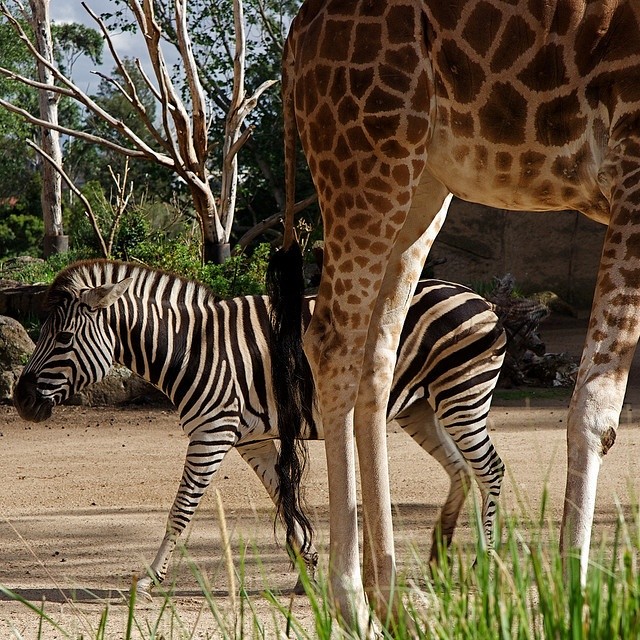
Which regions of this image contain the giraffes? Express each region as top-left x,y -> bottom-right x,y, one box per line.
265,0 -> 640,640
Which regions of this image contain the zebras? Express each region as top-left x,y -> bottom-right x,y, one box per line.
11,260 -> 509,608
